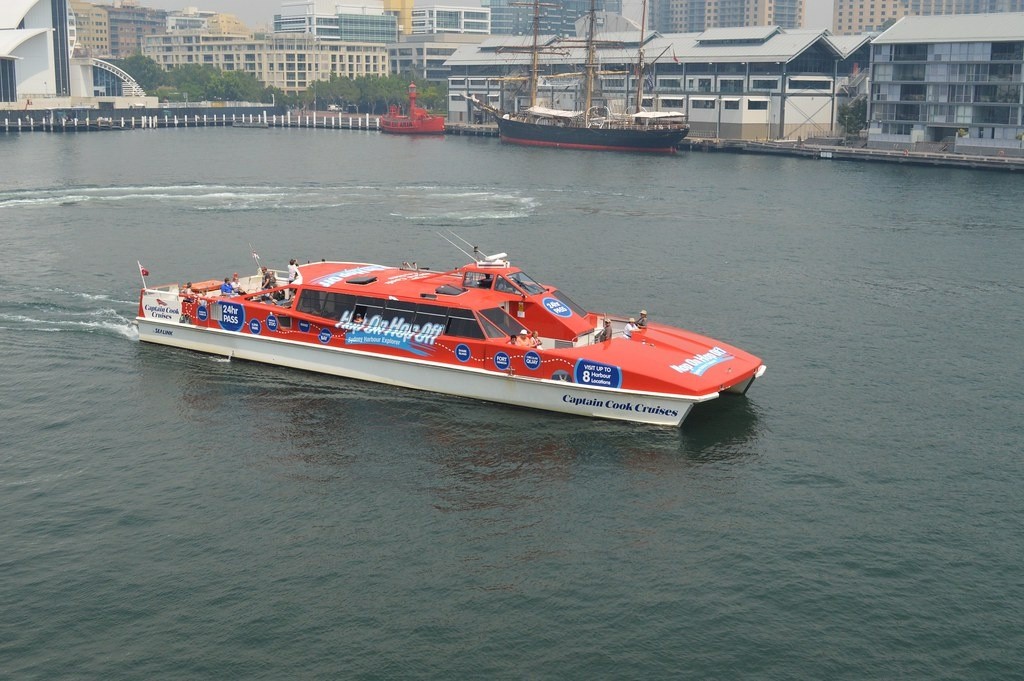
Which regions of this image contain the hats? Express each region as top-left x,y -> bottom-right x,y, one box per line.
520,329 -> 527,334
639,310 -> 647,315
605,319 -> 611,323
628,318 -> 636,323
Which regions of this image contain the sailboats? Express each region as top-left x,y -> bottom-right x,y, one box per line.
458,0 -> 692,153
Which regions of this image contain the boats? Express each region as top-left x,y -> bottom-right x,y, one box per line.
378,79 -> 445,135
134,251 -> 766,427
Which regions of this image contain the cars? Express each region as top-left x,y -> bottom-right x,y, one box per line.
327,105 -> 342,112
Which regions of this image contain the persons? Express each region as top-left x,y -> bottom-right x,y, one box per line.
288,259 -> 297,300
354,314 -> 363,323
259,267 -> 277,304
185,282 -> 195,300
221,278 -> 253,300
601,319 -> 612,341
503,112 -> 517,121
623,310 -> 647,338
483,274 -> 493,281
509,330 -> 542,349
